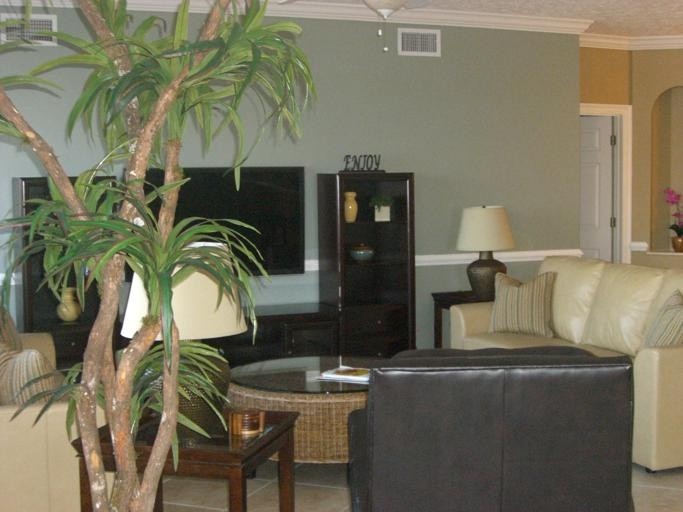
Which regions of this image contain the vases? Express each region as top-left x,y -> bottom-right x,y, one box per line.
672,237 -> 683,252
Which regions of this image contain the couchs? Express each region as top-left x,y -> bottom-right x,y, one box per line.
450,301 -> 683,474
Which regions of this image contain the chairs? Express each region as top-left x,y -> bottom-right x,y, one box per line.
0,332 -> 115,512
348,355 -> 635,512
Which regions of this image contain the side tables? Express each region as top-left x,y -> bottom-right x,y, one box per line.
72,409 -> 300,512
432,291 -> 482,349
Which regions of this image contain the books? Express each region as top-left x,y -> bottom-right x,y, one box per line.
316,365 -> 370,385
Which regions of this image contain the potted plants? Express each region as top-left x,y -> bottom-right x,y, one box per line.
368,195 -> 395,223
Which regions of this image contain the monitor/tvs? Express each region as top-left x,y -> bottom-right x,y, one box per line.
123,167 -> 304,282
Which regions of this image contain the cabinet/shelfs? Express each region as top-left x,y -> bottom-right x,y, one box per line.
118,303 -> 339,370
13,177 -> 116,365
318,156 -> 415,356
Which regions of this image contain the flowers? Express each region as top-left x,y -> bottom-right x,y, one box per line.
663,188 -> 683,236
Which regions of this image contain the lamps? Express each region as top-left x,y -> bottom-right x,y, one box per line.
364,0 -> 406,52
120,242 -> 248,444
456,205 -> 516,300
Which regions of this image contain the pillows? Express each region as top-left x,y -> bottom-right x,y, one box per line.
0,310 -> 70,406
390,347 -> 595,357
487,256 -> 683,357
